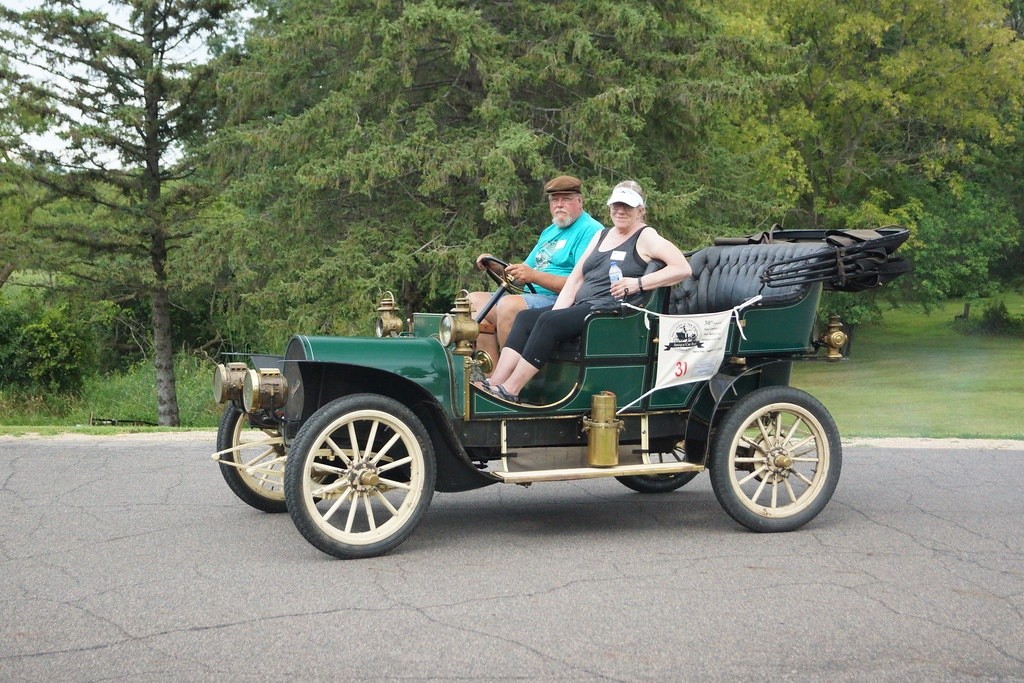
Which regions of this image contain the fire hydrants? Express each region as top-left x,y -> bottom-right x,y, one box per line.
820,312 -> 850,362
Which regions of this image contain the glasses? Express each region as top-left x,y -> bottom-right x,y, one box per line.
548,195 -> 579,204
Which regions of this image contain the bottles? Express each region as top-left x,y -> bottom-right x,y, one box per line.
609,262 -> 624,302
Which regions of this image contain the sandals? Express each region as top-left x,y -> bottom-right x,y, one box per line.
479,384 -> 518,404
470,380 -> 490,387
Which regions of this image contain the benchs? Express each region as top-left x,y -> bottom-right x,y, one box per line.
499,259 -> 671,364
669,242 -> 834,359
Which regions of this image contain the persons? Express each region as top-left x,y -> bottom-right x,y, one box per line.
465,176 -> 692,404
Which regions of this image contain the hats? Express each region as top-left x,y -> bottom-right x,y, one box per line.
544,176 -> 582,194
607,187 -> 643,207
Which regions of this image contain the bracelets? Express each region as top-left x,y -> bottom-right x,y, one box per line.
638,277 -> 644,294
